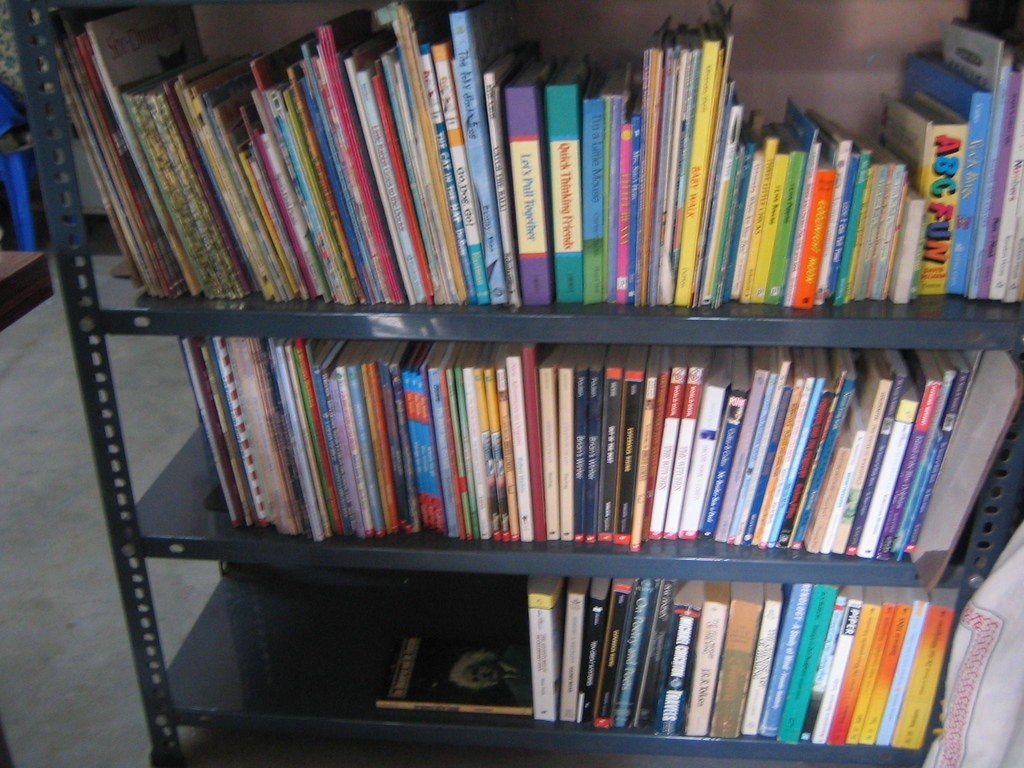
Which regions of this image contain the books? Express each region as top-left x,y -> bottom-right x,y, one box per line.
175,333 -> 1024,750
47,0 -> 1023,308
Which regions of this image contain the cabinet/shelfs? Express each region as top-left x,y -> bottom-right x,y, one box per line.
11,0 -> 1024,768
